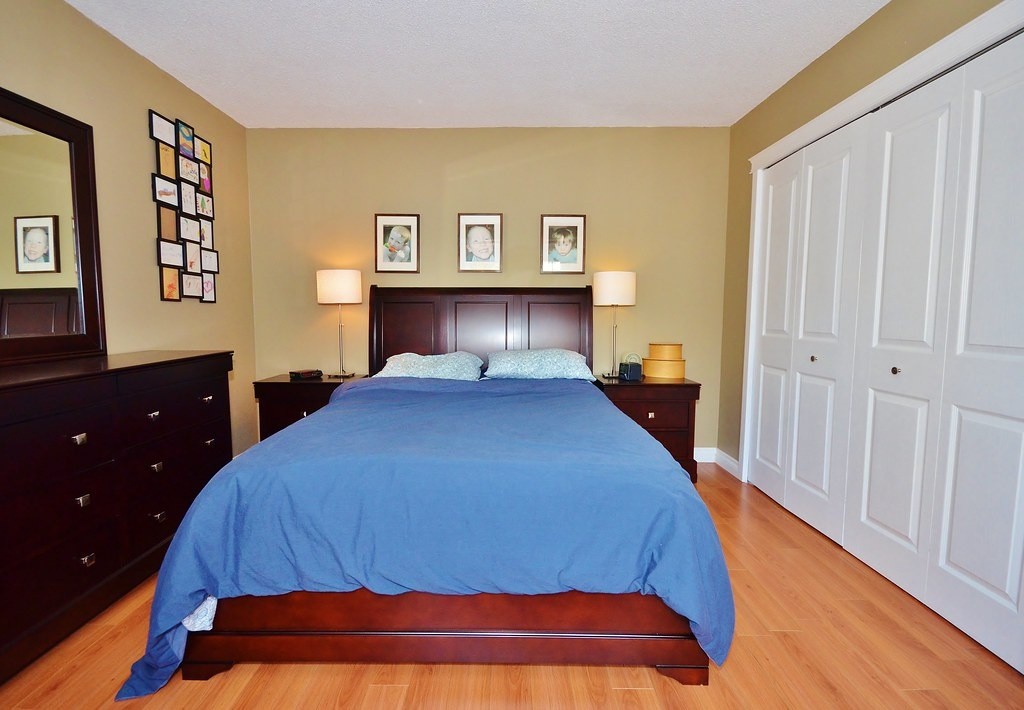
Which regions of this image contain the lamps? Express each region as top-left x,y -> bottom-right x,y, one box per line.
316,269 -> 363,377
593,271 -> 637,377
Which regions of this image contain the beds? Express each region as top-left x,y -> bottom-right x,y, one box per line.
114,284 -> 735,700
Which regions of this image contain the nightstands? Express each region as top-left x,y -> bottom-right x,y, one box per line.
252,375 -> 369,442
595,374 -> 702,483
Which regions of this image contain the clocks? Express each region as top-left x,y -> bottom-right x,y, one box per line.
621,352 -> 642,365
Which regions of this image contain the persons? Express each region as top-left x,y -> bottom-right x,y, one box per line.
383,225 -> 411,262
24,228 -> 49,263
466,226 -> 494,262
548,228 -> 577,265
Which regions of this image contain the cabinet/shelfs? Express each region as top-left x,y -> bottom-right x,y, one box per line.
0,349 -> 236,687
746,0 -> 1024,680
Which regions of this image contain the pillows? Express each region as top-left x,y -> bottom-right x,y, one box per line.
370,352 -> 484,381
484,348 -> 597,382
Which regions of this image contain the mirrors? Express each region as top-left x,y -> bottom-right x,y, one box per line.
0,86 -> 108,370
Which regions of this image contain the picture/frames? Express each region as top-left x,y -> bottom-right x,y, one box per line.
458,213 -> 503,272
375,213 -> 420,273
14,215 -> 60,274
148,109 -> 219,304
540,214 -> 586,273
